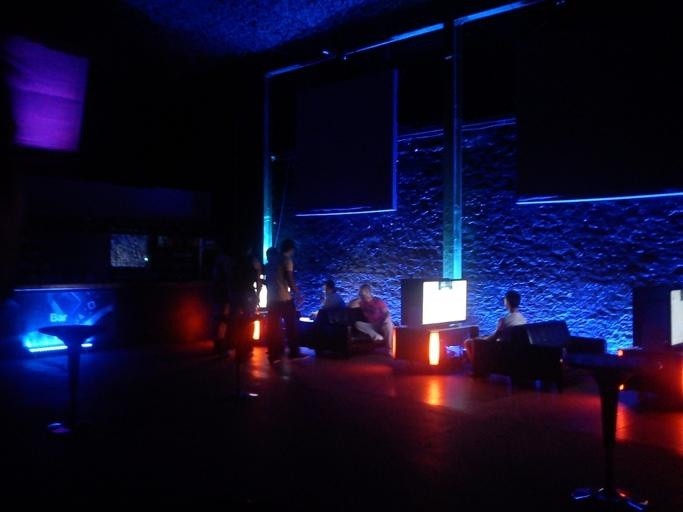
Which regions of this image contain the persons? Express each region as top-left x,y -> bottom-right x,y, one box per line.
209,237 -> 309,365
347,284 -> 394,359
473,287 -> 526,343
308,280 -> 347,324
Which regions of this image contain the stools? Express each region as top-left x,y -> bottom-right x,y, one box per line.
38,324 -> 103,428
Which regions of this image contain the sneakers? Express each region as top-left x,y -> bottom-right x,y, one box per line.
270,350 -> 309,364
384,350 -> 395,360
373,334 -> 384,342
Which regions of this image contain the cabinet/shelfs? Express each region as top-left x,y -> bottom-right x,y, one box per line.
394,324 -> 480,375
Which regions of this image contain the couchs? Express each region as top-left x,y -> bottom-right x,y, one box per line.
314,308 -> 385,360
473,320 -> 612,396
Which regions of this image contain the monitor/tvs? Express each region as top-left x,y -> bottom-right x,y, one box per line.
668,287 -> 683,347
420,278 -> 468,327
